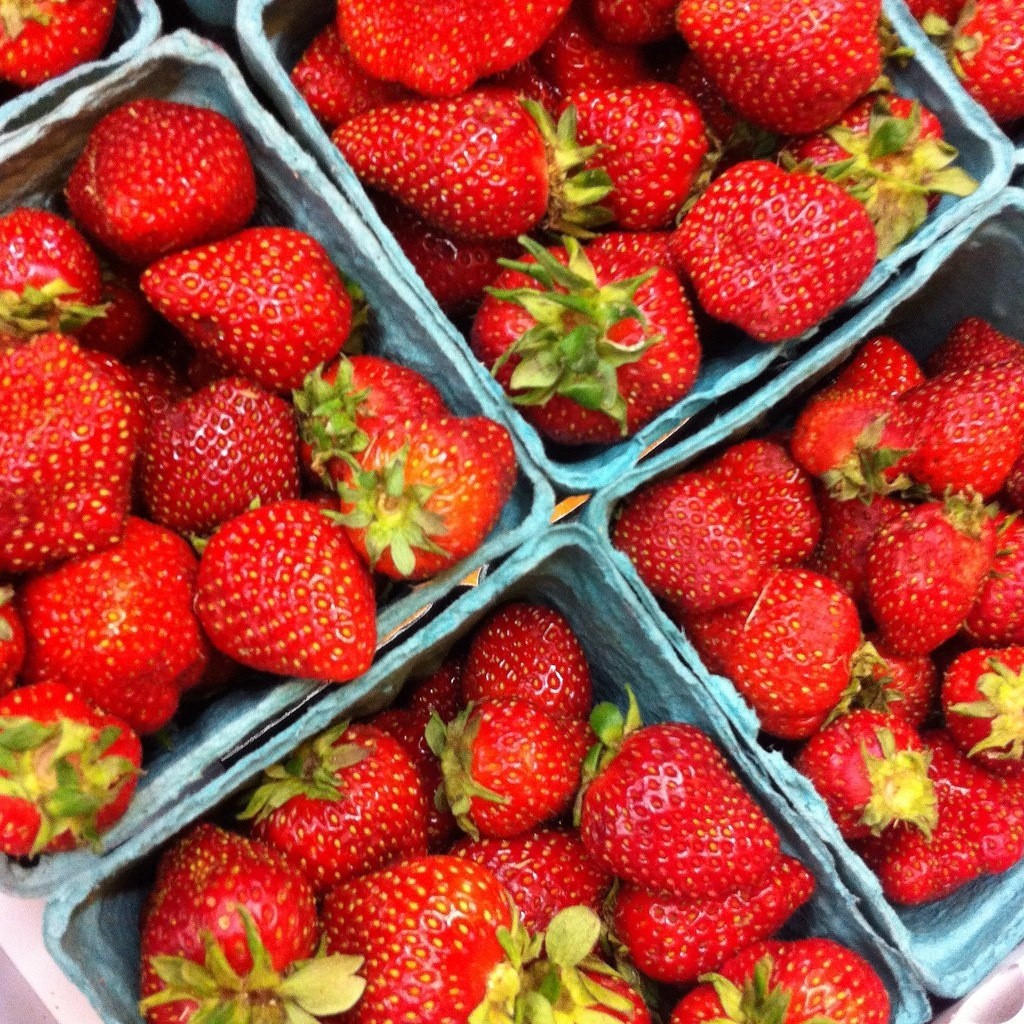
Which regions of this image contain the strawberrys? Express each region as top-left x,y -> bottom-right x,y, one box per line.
0,0 -> 1024,1024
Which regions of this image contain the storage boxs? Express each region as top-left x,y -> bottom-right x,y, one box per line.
1,1 -> 1024,1024
